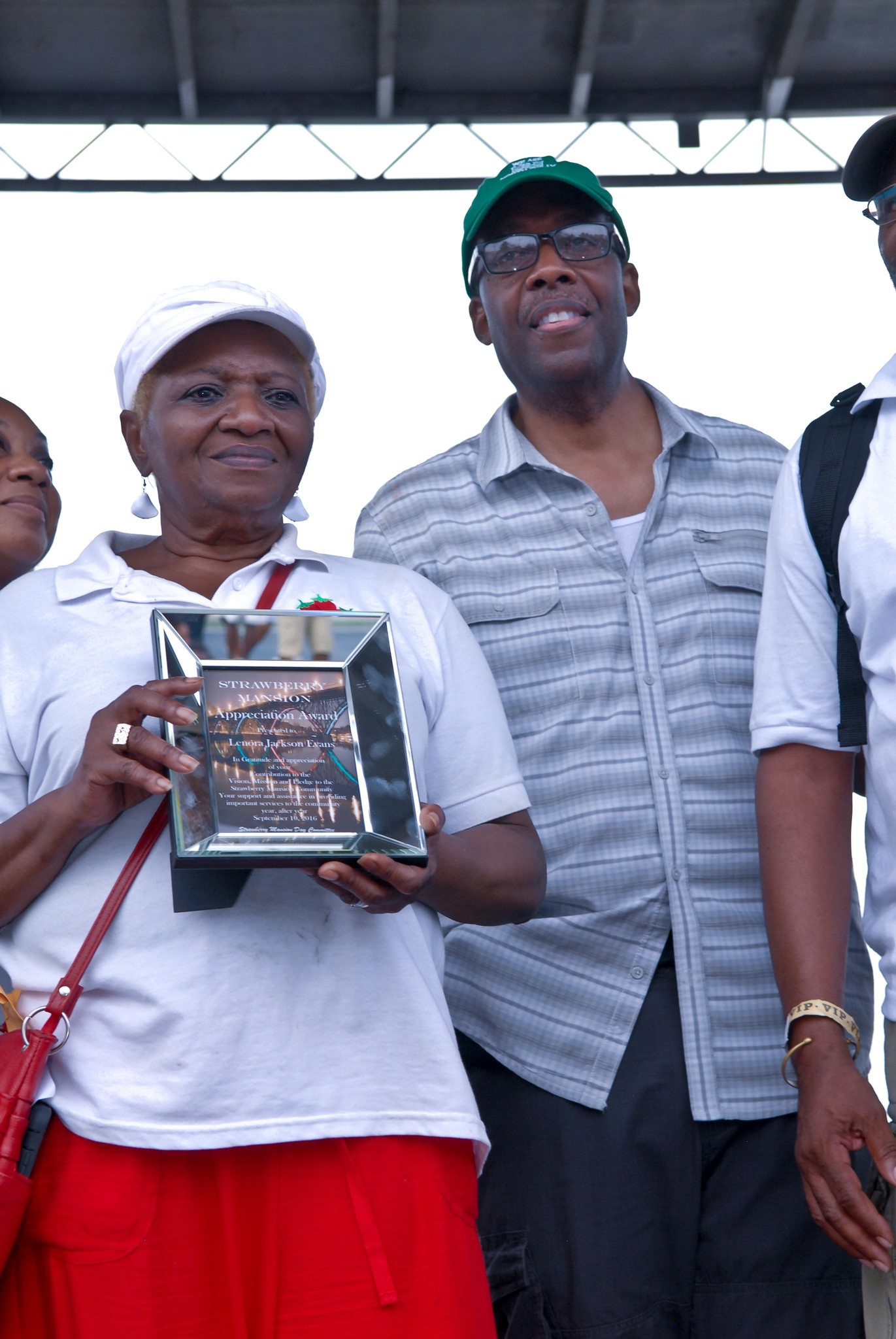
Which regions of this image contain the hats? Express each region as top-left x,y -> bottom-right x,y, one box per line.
461,157 -> 630,297
841,114 -> 896,203
117,281 -> 326,415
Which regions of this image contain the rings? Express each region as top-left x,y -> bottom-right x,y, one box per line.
112,723 -> 132,754
351,900 -> 369,908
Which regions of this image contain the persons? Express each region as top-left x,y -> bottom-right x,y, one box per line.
754,109 -> 896,1339
0,396 -> 61,589
352,156 -> 867,1339
0,286 -> 545,1339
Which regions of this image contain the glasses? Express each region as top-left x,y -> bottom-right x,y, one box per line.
466,220 -> 628,290
862,181 -> 896,227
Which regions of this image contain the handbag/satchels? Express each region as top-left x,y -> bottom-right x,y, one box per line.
0,1010 -> 69,1273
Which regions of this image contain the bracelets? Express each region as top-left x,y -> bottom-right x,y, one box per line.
783,999 -> 860,1060
782,1037 -> 856,1089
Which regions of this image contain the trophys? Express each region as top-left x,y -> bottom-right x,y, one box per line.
150,607 -> 429,870
